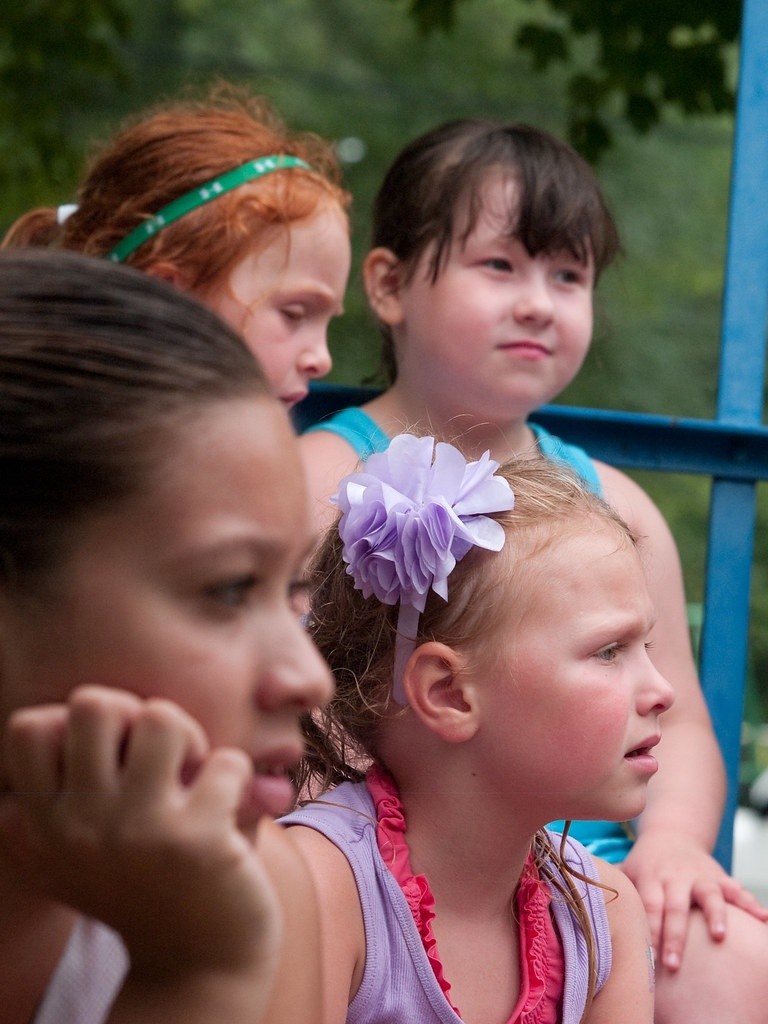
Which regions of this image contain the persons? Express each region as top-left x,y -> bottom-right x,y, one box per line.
297,121 -> 766,1023
0,87 -> 351,418
2,245 -> 333,1023
270,434 -> 677,1024
